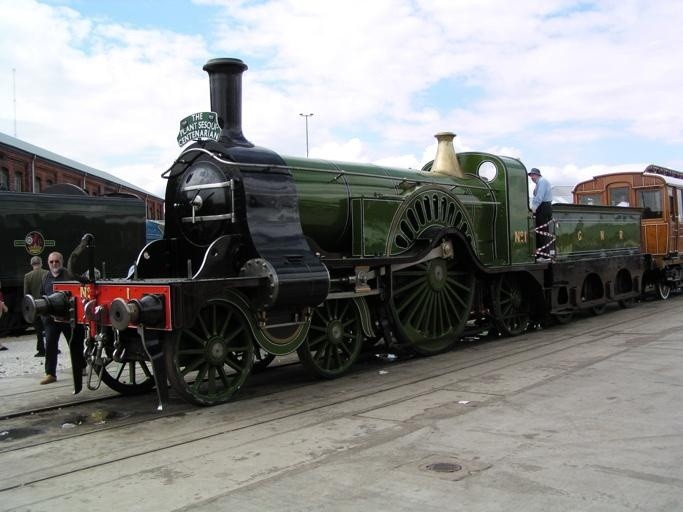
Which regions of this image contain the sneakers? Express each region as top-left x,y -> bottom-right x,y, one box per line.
537,256 -> 550,262
35,348 -> 60,356
0,346 -> 8,350
40,374 -> 56,384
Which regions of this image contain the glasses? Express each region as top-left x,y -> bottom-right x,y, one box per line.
49,260 -> 60,263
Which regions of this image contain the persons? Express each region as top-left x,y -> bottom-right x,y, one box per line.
527,167 -> 552,262
25,255 -> 62,356
616,195 -> 629,207
38,251 -> 88,386
81,263 -> 100,281
0,290 -> 9,351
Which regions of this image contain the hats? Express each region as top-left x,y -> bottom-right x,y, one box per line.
527,168 -> 542,176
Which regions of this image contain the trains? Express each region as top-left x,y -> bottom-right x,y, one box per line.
21,54 -> 683,410
